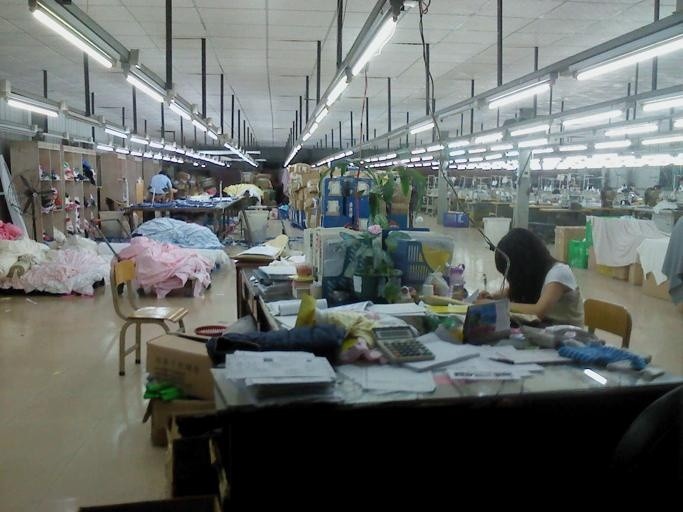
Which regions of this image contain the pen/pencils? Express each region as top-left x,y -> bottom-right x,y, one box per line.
483,273 -> 487,295
488,357 -> 514,364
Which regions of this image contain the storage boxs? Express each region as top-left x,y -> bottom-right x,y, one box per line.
553,225 -> 628,280
287,163 -> 320,229
142,331 -> 214,447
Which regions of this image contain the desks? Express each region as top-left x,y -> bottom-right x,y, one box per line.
120,196 -> 245,243
419,187 -> 652,245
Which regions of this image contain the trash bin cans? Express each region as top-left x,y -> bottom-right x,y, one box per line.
194,326 -> 228,339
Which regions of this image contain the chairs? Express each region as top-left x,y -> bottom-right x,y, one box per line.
109,257 -> 188,377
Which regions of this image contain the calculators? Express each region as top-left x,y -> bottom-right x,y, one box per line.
370,325 -> 436,362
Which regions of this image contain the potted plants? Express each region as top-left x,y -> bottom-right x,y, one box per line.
316,159 -> 426,303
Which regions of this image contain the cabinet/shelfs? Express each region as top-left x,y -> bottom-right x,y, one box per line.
9,140 -> 160,244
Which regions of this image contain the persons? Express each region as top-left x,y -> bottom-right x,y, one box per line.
599,181 -> 663,209
146,170 -> 172,202
153,174 -> 178,218
472,226 -> 585,331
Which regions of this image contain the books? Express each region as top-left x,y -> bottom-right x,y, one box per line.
304,227 -> 363,284
404,332 -> 478,374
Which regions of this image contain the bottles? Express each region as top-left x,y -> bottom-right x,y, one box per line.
448,262 -> 464,303
